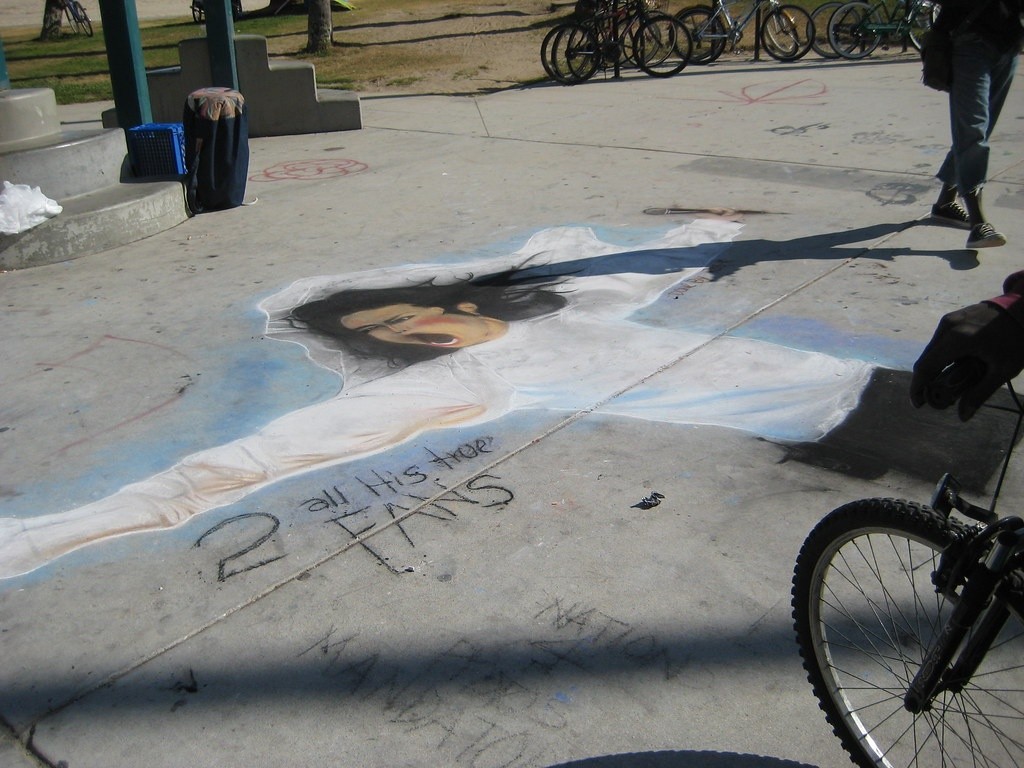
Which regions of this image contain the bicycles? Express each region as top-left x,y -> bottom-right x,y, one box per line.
537,0 -> 943,86
60,0 -> 93,37
188,0 -> 243,24
790,344 -> 1024,768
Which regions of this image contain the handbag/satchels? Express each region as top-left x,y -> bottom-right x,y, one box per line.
921,22 -> 953,92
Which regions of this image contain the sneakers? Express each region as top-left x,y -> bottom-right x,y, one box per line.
930,201 -> 970,227
966,223 -> 1006,248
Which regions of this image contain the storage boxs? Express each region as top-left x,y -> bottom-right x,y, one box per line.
126,121 -> 185,174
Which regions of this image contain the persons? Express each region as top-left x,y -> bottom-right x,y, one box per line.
931,0 -> 1024,249
911,270 -> 1024,423
